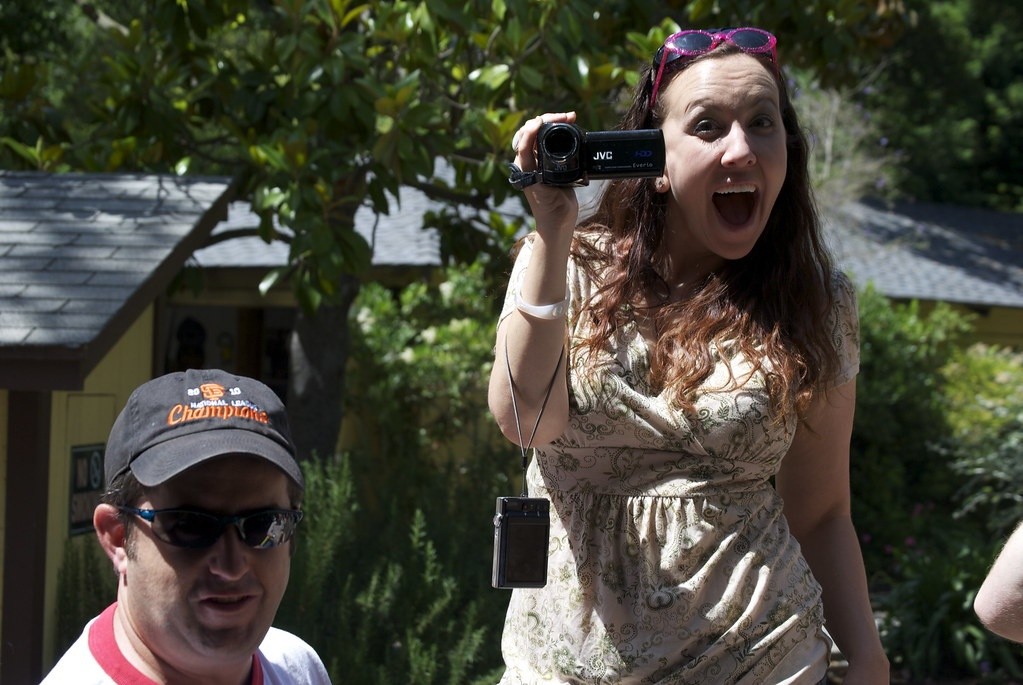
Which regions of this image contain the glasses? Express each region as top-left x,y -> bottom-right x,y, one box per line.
647,27 -> 780,119
112,504 -> 305,552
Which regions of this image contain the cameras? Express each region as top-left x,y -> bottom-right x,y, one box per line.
491,498 -> 550,588
535,122 -> 667,187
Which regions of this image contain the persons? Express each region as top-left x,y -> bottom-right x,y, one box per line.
481,24 -> 893,685
975,517 -> 1022,646
34,364 -> 335,685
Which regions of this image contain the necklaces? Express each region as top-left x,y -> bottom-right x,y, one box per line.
644,282 -> 669,399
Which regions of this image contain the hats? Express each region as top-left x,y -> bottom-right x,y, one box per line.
103,368 -> 307,496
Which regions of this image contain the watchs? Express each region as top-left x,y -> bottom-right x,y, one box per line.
512,266 -> 572,319
